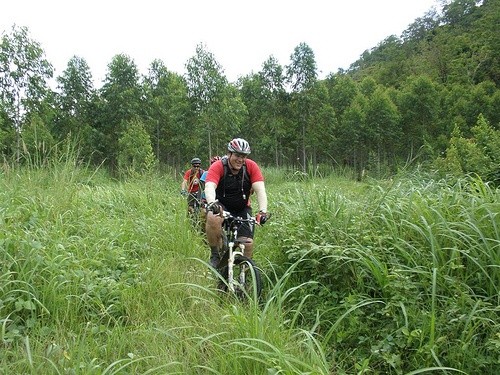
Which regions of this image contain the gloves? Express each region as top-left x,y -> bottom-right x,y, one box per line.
254,212 -> 268,225
207,203 -> 225,215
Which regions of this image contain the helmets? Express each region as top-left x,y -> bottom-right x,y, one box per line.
211,156 -> 221,163
191,158 -> 201,164
228,138 -> 251,155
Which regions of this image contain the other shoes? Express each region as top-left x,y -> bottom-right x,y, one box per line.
209,250 -> 221,267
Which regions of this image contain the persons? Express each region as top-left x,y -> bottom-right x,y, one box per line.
182,158 -> 205,227
205,139 -> 267,268
200,155 -> 221,243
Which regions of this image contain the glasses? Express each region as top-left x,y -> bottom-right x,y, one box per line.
193,166 -> 199,167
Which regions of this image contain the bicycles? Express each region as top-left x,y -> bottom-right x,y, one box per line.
186,191 -> 262,299
